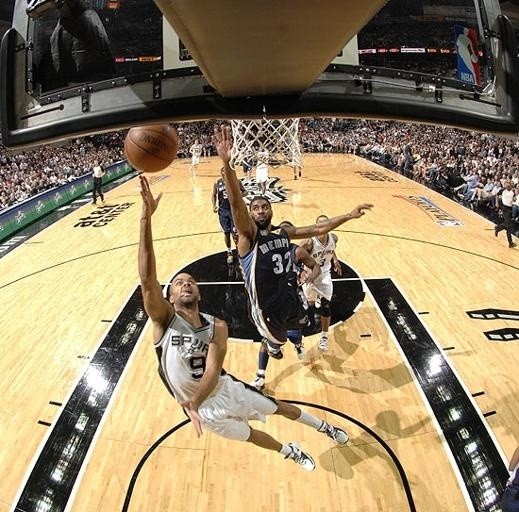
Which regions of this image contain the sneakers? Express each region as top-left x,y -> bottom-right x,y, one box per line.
317,420 -> 349,445
509,242 -> 517,248
294,335 -> 305,359
92,199 -> 97,204
319,335 -> 329,352
299,171 -> 301,176
261,338 -> 283,359
495,227 -> 498,237
294,174 -> 297,180
250,374 -> 266,391
314,298 -> 321,308
227,251 -> 233,264
283,441 -> 315,470
101,193 -> 104,203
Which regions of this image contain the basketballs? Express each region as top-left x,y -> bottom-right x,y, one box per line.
125,123 -> 178,171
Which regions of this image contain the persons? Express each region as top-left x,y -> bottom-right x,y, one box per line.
1,129 -> 130,212
136,174 -> 349,472
169,119 -> 367,167
359,119 -> 519,248
210,123 -> 373,392
